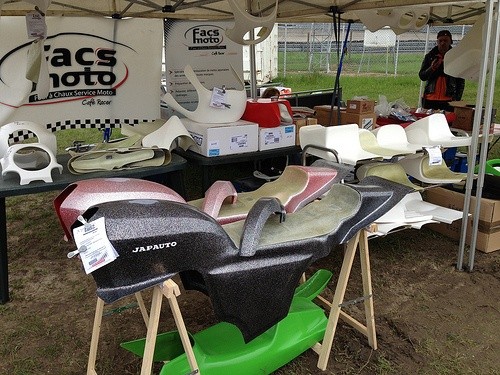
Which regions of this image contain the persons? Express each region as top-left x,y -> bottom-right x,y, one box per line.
262,88 -> 280,100
418,29 -> 466,112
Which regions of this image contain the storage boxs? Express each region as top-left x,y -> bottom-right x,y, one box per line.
423,183 -> 500,254
451,107 -> 497,131
180,118 -> 318,158
314,99 -> 377,131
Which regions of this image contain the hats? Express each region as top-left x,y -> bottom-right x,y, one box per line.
437,29 -> 454,44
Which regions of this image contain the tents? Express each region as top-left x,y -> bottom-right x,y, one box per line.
0,0 -> 500,24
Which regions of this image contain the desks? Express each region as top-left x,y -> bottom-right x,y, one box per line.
0,144 -> 304,305
